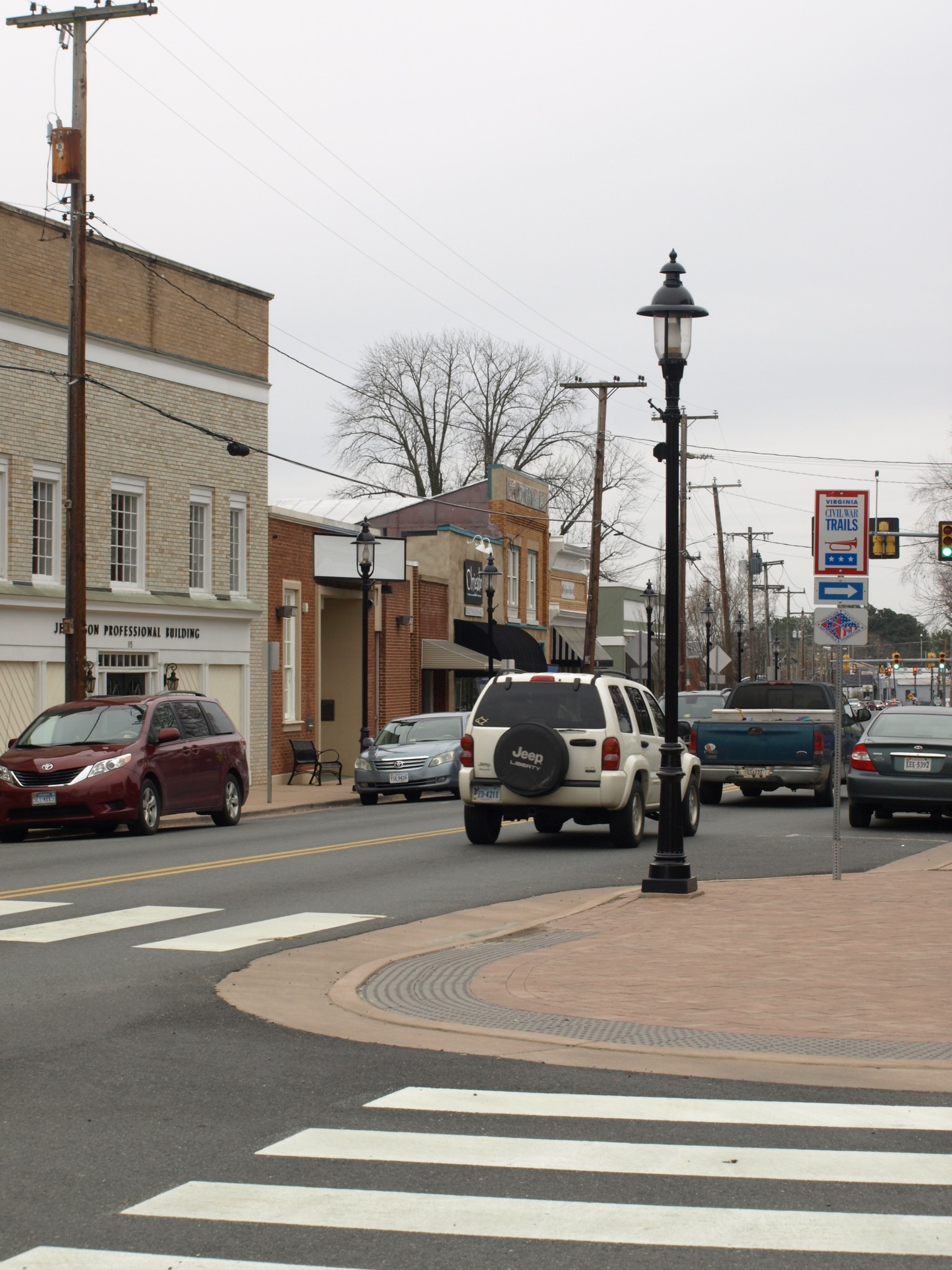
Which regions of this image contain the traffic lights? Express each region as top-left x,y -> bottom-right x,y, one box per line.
937,521 -> 952,561
939,652 -> 946,669
885,669 -> 888,676
888,668 -> 891,676
913,667 -> 917,675
927,653 -> 936,668
892,653 -> 900,670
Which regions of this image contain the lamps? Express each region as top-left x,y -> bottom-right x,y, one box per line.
275,605 -> 298,618
397,616 -> 414,625
163,663 -> 180,691
467,535 -> 494,556
83,661 -> 97,693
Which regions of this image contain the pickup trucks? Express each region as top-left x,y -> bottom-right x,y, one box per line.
690,680 -> 873,809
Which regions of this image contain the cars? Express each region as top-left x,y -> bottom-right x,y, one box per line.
885,698 -> 902,707
848,698 -> 862,709
355,711 -> 473,806
845,707 -> 952,829
866,700 -> 886,710
1,689 -> 251,836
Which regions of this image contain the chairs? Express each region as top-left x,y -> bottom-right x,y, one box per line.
287,739 -> 342,786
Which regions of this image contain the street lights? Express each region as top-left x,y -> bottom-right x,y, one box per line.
730,611 -> 747,683
350,515 -> 383,761
699,598 -> 717,691
822,646 -> 832,684
475,552 -> 504,682
639,579 -> 659,691
634,249 -> 714,898
771,634 -> 783,680
920,634 -> 924,668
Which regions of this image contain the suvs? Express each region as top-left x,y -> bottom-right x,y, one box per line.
458,668 -> 704,847
657,688 -> 734,757
